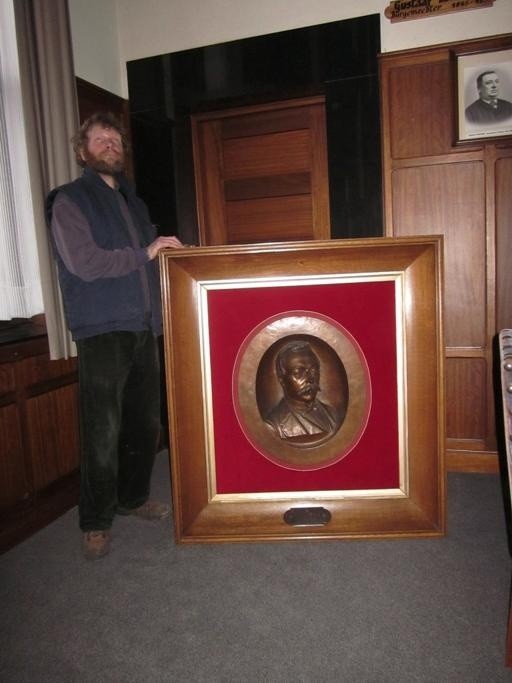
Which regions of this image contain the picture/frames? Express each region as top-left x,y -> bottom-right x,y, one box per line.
448,34 -> 512,148
159,236 -> 446,545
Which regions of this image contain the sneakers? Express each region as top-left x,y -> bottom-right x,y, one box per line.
118,502 -> 170,519
83,531 -> 110,558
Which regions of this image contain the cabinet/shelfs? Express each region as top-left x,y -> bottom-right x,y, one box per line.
1,314 -> 97,557
372,35 -> 512,474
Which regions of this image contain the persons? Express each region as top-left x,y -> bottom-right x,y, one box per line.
464,71 -> 512,122
261,342 -> 343,435
45,109 -> 184,557
